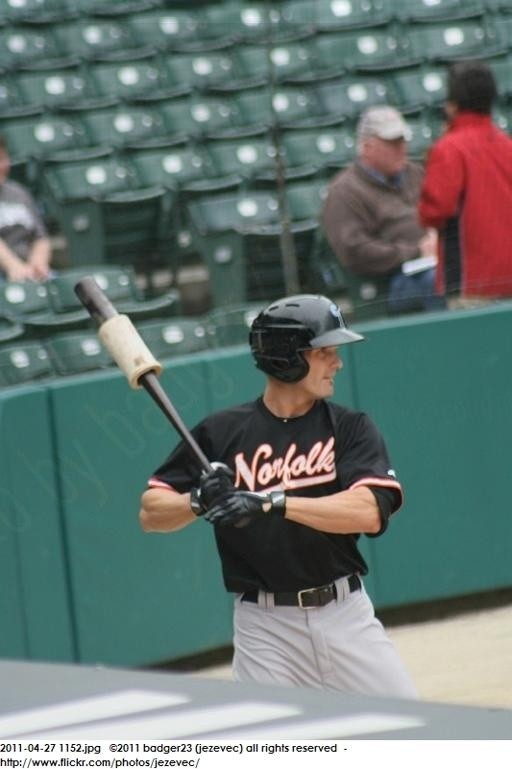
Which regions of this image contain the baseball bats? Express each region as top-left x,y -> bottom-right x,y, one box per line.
73,276 -> 252,529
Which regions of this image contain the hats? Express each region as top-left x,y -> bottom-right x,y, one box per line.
359,104 -> 412,143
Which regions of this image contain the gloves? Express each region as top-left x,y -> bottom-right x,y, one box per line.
203,487 -> 289,527
190,461 -> 238,519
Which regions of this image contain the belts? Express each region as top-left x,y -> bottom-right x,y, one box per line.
236,572 -> 363,607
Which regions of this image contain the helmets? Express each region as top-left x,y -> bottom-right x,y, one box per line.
250,296 -> 364,384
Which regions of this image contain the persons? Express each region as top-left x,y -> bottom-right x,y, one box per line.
0,140 -> 60,286
416,64 -> 512,301
316,102 -> 451,311
137,295 -> 423,698
